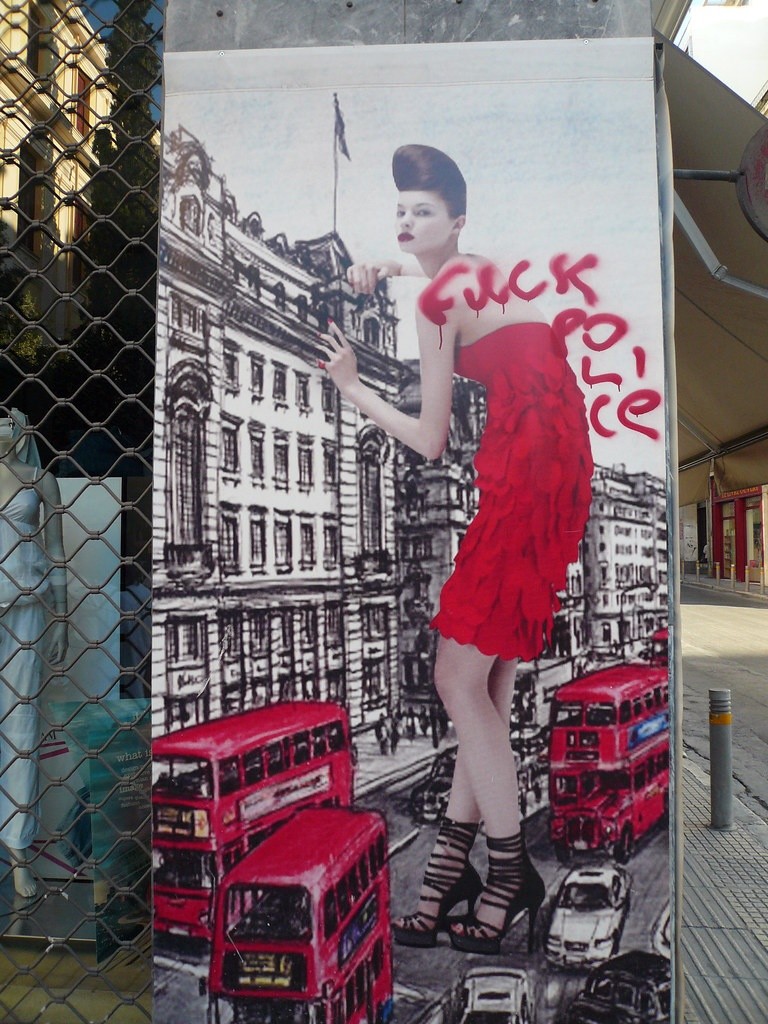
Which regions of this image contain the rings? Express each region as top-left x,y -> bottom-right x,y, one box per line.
319,362 -> 325,369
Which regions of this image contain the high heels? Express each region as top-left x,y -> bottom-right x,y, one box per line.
391,815 -> 486,947
448,825 -> 546,955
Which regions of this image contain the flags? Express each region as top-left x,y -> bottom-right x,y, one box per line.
334,109 -> 350,160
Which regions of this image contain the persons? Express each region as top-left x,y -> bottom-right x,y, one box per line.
377,705 -> 430,756
0,434 -> 67,895
319,146 -> 592,951
